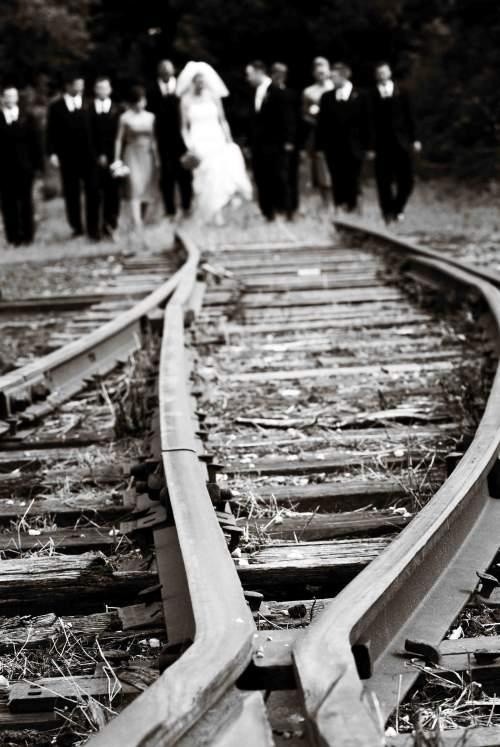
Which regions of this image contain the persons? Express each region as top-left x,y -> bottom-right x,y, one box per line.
1,55 -> 423,247
